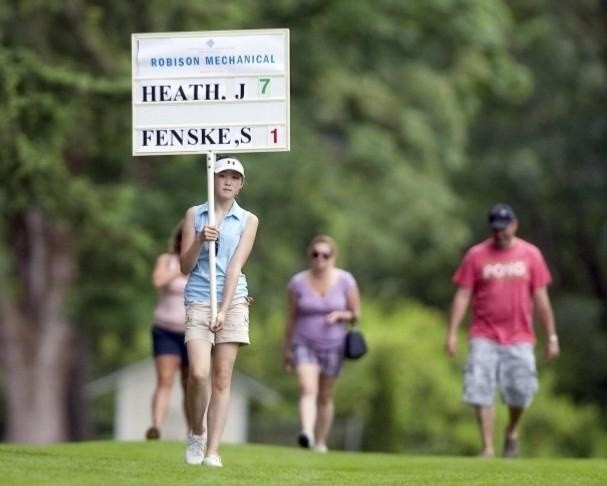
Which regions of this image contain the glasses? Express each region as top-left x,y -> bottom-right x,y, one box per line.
313,251 -> 333,261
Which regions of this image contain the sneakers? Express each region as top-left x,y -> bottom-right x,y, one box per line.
502,425 -> 519,455
146,426 -> 160,440
184,424 -> 208,466
298,431 -> 315,448
201,454 -> 223,467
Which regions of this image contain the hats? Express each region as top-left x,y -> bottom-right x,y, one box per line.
214,157 -> 248,179
488,203 -> 516,230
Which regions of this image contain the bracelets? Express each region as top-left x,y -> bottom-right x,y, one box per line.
545,336 -> 559,343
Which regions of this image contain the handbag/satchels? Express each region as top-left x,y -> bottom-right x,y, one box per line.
344,330 -> 367,358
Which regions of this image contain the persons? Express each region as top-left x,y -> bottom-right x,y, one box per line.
282,234 -> 359,454
179,153 -> 258,468
146,219 -> 190,439
445,202 -> 560,457
